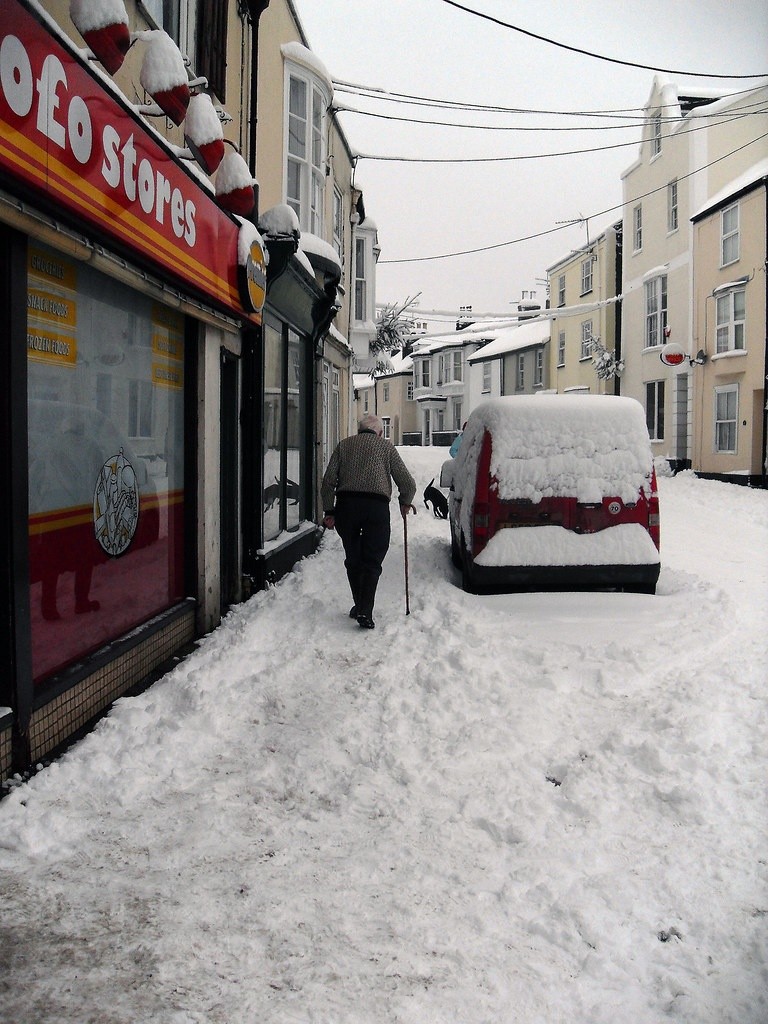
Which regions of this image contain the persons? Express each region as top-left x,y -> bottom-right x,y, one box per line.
449,422 -> 467,459
30,414 -> 104,621
320,417 -> 416,626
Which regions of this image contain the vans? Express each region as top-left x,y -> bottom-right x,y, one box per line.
439,393 -> 660,595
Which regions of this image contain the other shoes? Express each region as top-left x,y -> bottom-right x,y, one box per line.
351,606 -> 362,617
356,608 -> 375,629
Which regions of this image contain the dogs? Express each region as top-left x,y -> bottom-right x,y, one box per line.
422,478 -> 449,519
263,476 -> 299,514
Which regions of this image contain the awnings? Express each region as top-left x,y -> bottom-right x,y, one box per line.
466,321 -> 550,365
